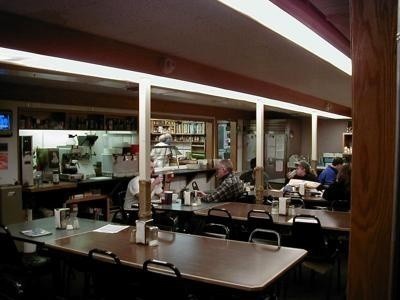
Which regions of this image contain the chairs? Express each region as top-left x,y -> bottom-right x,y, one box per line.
0,162 -> 351,300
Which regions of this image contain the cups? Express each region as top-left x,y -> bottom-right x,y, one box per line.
164,191 -> 173,204
289,169 -> 297,178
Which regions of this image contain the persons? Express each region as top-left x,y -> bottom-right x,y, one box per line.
319,157 -> 342,185
123,167 -> 174,230
322,164 -> 351,210
196,160 -> 250,204
285,159 -> 318,184
151,134 -> 183,167
240,158 -> 269,190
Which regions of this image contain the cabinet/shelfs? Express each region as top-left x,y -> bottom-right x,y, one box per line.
341,133 -> 353,161
150,119 -> 213,159
243,118 -> 300,181
18,110 -> 138,133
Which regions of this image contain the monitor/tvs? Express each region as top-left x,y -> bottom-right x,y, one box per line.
0,109 -> 13,137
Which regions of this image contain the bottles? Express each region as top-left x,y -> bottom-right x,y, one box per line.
147,226 -> 159,247
271,201 -> 278,214
69,203 -> 79,230
288,205 -> 296,217
191,193 -> 201,206
53,170 -> 59,184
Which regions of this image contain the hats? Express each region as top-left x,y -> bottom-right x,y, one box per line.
294,160 -> 310,169
